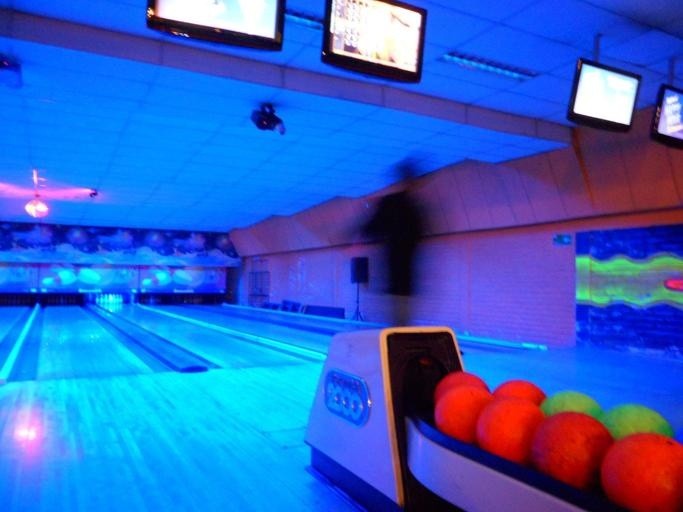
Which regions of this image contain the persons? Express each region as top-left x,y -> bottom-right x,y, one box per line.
364,158 -> 430,330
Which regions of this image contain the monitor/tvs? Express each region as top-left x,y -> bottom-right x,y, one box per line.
649,84 -> 682,148
319,0 -> 428,85
566,57 -> 643,132
145,0 -> 286,51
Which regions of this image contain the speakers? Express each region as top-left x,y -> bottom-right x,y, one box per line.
351,257 -> 369,283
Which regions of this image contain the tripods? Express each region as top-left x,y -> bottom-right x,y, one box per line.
351,285 -> 364,320
